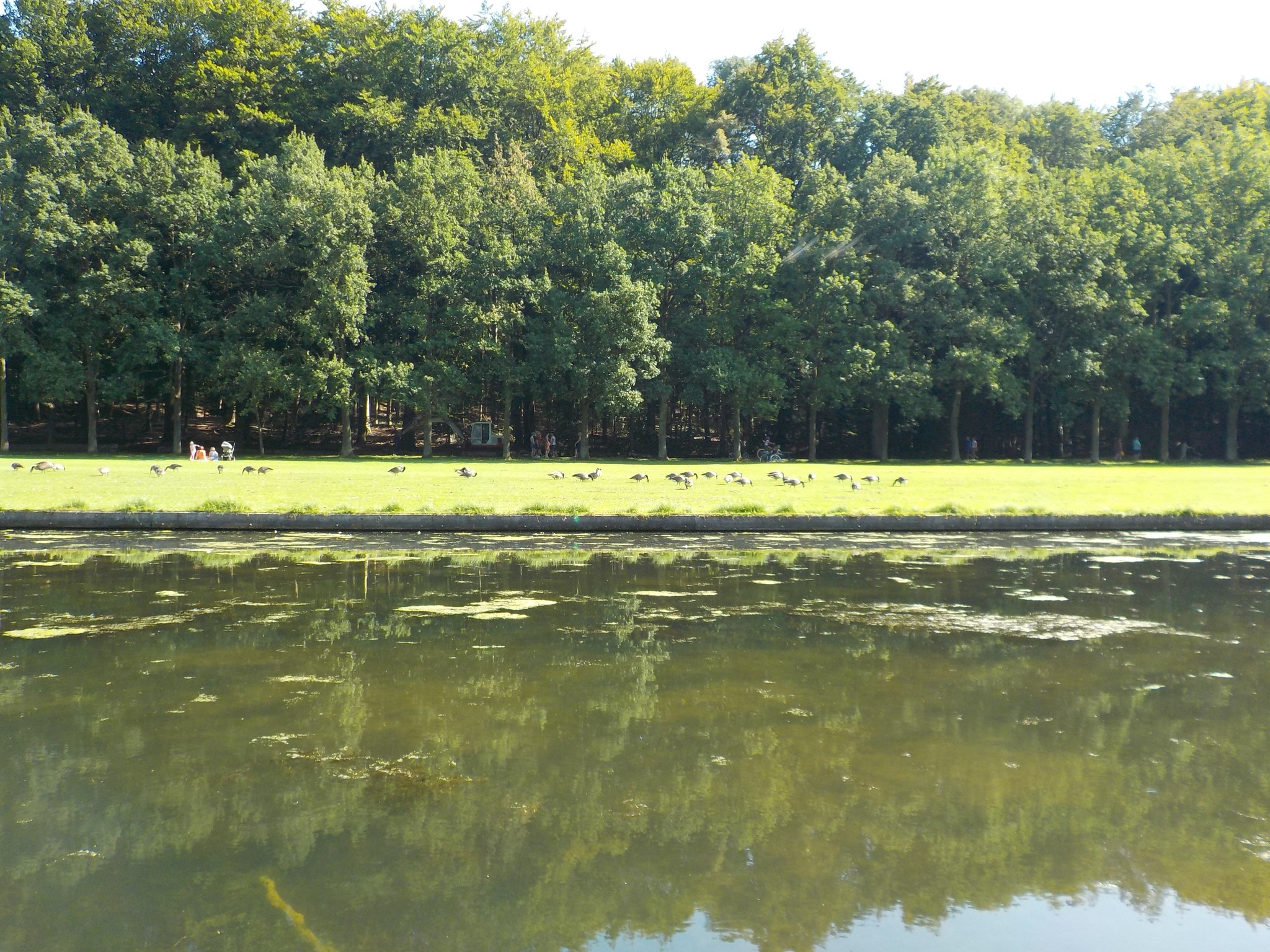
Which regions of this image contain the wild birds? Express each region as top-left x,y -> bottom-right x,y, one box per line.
766,472 -> 805,489
588,467 -> 602,482
833,474 -> 854,483
217,465 -> 225,473
242,466 -> 257,475
629,473 -> 649,484
150,463 -> 183,477
454,467 -> 477,479
892,477 -> 909,486
861,475 -> 881,485
852,482 -> 861,492
387,465 -> 406,476
98,467 -> 110,476
724,471 -> 754,488
547,471 -> 564,480
701,471 -> 718,480
11,461 -> 65,473
664,471 -> 698,490
808,473 -> 816,482
572,473 -> 593,483
258,466 -> 273,476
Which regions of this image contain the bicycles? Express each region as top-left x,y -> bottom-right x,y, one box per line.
729,450 -> 749,460
756,445 -> 783,462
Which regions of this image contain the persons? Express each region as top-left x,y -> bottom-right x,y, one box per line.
572,432 -> 582,458
195,445 -> 207,462
208,447 -> 218,460
1114,437 -> 1123,460
1133,436 -> 1141,461
965,436 -> 973,460
971,437 -> 979,459
550,432 -> 558,458
544,432 -> 551,458
530,431 -> 542,458
764,436 -> 776,454
189,441 -> 200,461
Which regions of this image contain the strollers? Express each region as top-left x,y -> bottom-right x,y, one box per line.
221,441 -> 236,461
556,439 -> 573,457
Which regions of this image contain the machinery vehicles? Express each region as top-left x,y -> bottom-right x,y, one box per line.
392,416 -> 504,457
